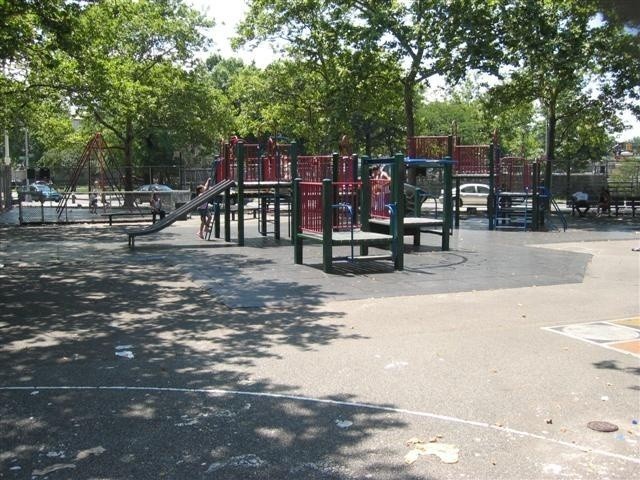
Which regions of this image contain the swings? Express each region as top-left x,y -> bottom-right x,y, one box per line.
86,135 -> 112,211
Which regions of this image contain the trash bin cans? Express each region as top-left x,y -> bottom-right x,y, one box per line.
175,203 -> 187,220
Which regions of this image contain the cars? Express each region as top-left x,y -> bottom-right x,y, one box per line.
17,183 -> 61,203
133,184 -> 172,204
438,183 -> 524,208
191,184 -> 248,207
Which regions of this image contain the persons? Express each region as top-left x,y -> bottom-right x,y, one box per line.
371,165 -> 391,214
197,178 -> 212,239
148,193 -> 165,219
498,184 -> 514,225
594,186 -> 610,217
571,186 -> 591,218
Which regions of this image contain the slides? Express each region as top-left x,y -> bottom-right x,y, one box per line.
124,178 -> 234,236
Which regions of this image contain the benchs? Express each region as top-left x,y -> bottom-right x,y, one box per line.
100,211 -> 170,225
230,208 -> 258,220
566,191 -> 640,218
